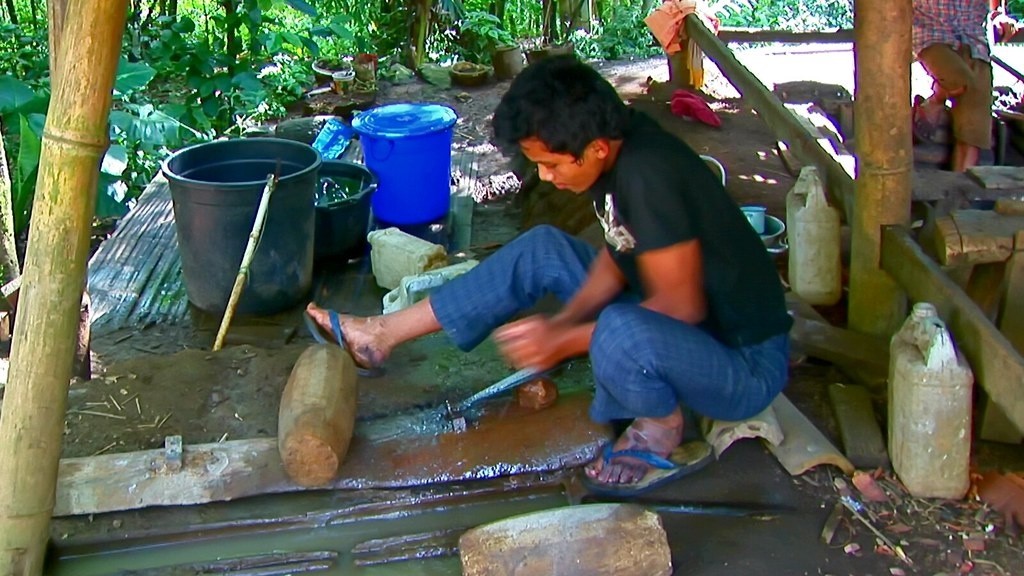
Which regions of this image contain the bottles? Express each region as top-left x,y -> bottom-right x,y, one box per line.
889,301 -> 974,499
786,166 -> 841,302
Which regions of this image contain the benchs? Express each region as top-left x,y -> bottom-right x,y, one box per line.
935,209 -> 1024,446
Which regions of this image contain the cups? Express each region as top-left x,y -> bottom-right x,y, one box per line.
741,207 -> 766,234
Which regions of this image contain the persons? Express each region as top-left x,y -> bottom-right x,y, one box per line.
303,54 -> 794,496
911,0 -> 1019,176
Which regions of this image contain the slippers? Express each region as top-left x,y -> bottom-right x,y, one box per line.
912,94 -> 935,144
582,441 -> 715,496
303,308 -> 388,378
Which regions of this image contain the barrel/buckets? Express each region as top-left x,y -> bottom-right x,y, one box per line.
315,160 -> 377,260
332,71 -> 355,94
162,138 -> 323,316
351,103 -> 458,226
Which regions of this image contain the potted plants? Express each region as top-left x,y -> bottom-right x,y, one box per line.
310,13 -> 379,93
462,11 -> 522,79
526,38 -> 574,66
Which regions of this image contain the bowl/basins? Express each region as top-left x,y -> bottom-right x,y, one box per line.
758,214 -> 785,247
767,241 -> 789,253
